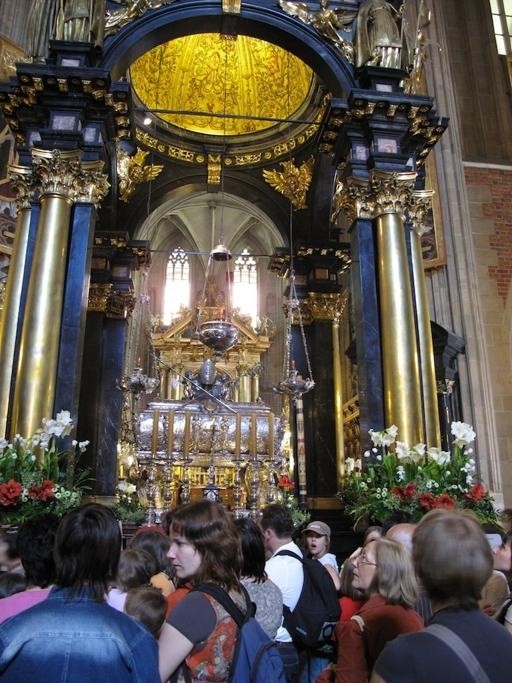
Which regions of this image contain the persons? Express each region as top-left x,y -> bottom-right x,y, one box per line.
1,502 -> 511,682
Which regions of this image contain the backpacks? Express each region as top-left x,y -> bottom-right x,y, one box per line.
273,549 -> 341,648
188,580 -> 288,682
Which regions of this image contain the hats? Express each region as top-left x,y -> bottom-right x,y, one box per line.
301,520 -> 331,537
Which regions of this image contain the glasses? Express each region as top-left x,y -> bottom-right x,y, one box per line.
359,553 -> 376,565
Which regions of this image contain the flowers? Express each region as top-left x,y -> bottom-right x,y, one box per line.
277,476 -> 310,526
115,481 -> 149,523
339,421 -> 499,538
1,410 -> 97,521
